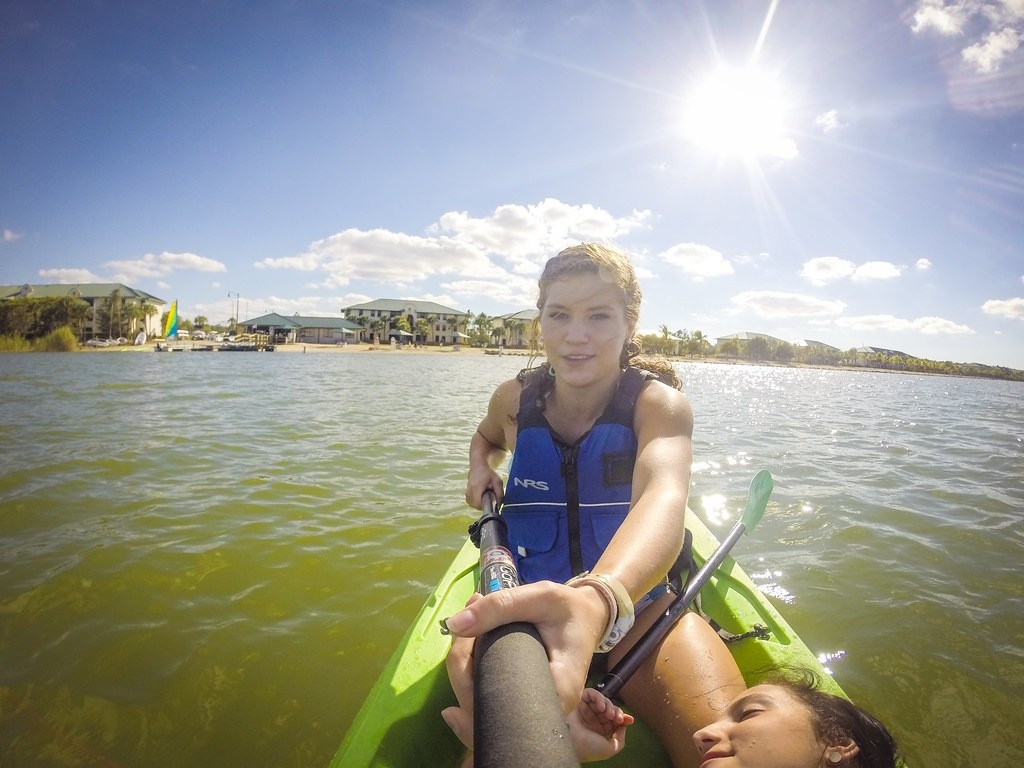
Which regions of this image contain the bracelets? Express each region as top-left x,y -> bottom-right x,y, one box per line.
563,577 -> 618,650
561,572 -> 636,654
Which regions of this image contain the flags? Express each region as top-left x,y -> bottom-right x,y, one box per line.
162,299 -> 179,338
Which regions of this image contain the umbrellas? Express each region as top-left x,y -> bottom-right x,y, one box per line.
447,331 -> 471,347
333,327 -> 356,347
386,330 -> 414,345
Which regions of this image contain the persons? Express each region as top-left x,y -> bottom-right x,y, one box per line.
456,662 -> 900,767
441,240 -> 749,766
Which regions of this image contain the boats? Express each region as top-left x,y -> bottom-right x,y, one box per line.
328,484 -> 909,768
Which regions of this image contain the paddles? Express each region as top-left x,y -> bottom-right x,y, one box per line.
466,487 -> 586,768
591,467 -> 774,702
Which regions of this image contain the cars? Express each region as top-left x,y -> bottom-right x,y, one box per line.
86,337 -> 127,347
177,329 -> 220,340
